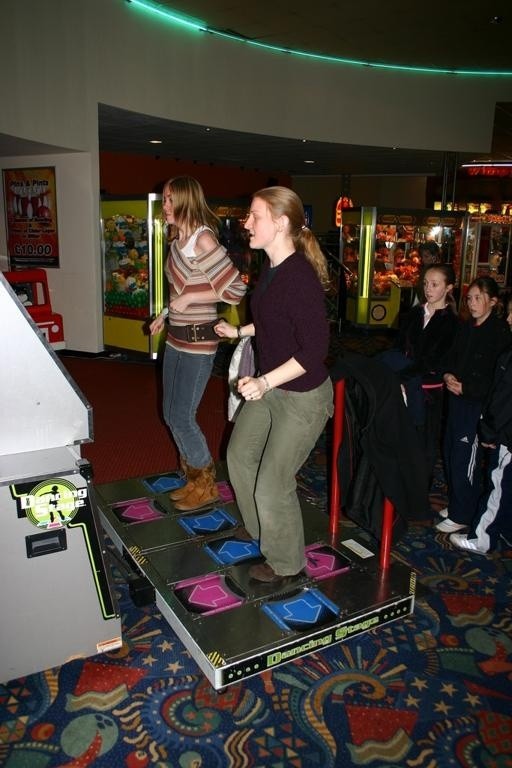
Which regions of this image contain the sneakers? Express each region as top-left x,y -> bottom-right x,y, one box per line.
435,508 -> 467,533
449,532 -> 491,556
248,562 -> 285,583
233,526 -> 253,542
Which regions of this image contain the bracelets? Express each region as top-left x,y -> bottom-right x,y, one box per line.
261,373 -> 271,393
235,325 -> 244,338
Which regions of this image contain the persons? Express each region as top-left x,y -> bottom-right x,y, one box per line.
434,276 -> 508,533
212,184 -> 336,583
148,174 -> 249,513
411,241 -> 459,316
388,264 -> 463,490
449,293 -> 511,555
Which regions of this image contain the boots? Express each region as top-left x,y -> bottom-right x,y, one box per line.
168,456 -> 196,501
174,455 -> 220,511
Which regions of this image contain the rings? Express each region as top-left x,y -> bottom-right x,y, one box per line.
249,394 -> 255,400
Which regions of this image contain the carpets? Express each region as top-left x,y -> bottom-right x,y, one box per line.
1,439 -> 510,768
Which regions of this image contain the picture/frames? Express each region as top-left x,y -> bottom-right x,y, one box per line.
2,166 -> 59,269
